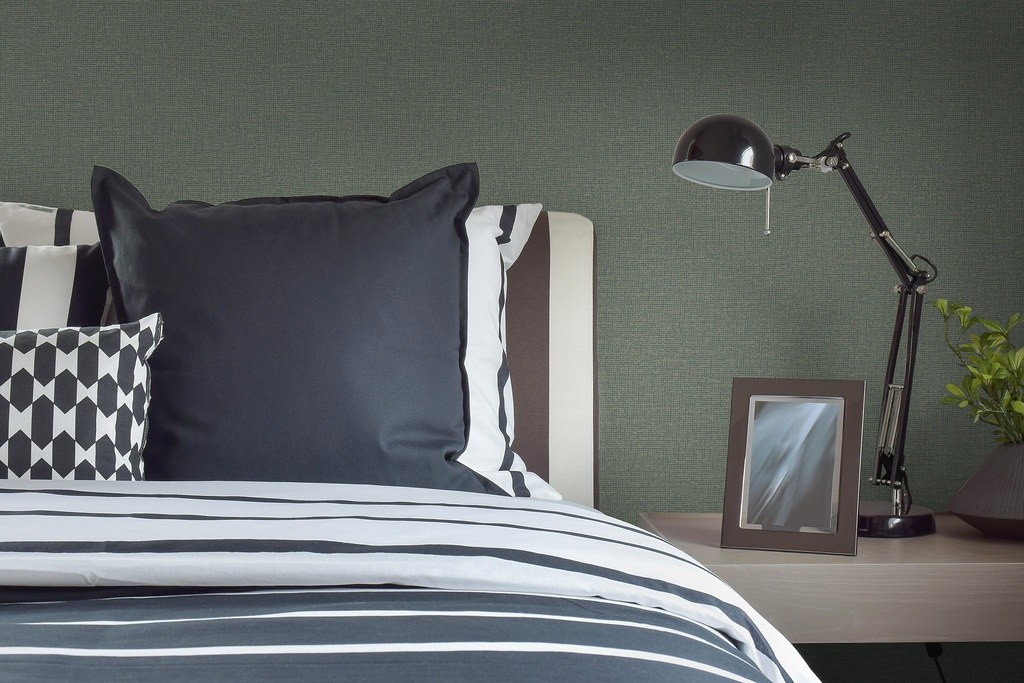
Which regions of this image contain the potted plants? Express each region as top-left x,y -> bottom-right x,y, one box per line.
923,298 -> 1024,542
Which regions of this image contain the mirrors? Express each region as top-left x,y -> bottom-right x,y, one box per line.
720,377 -> 868,556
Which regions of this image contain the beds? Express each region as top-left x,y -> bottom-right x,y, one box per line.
0,202 -> 822,683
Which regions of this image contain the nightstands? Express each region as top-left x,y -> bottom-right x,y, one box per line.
638,499 -> 1024,645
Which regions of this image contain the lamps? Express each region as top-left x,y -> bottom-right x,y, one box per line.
672,113 -> 938,539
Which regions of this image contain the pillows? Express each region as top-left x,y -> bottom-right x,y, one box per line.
1,311 -> 165,481
0,203 -> 562,501
90,162 -> 511,496
0,241 -> 119,331
